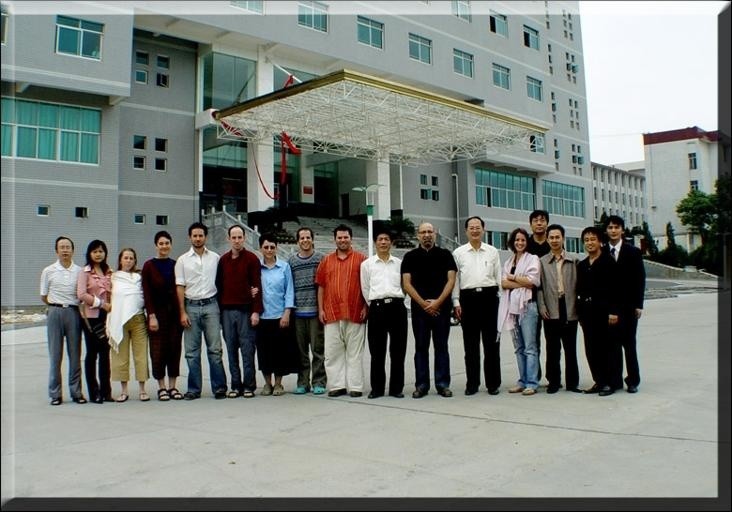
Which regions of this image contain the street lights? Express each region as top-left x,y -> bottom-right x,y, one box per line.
352,184 -> 388,260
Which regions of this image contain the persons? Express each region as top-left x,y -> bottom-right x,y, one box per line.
601,215 -> 645,394
173,219 -> 228,400
525,208 -> 552,383
285,224 -> 328,397
574,228 -> 622,398
105,248 -> 151,403
534,223 -> 582,395
215,225 -> 263,398
314,223 -> 369,398
451,215 -> 503,397
249,231 -> 294,395
358,227 -> 408,400
401,220 -> 459,400
38,234 -> 85,408
140,230 -> 186,400
500,228 -> 543,397
76,241 -> 115,405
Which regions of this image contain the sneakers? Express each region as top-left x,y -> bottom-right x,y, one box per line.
214,385 -> 403,400
411,387 -> 637,399
50,387 -> 200,406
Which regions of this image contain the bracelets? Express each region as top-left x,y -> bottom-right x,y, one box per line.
512,274 -> 518,280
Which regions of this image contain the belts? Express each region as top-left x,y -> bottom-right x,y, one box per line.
379,298 -> 399,303
48,301 -> 77,309
461,287 -> 497,293
